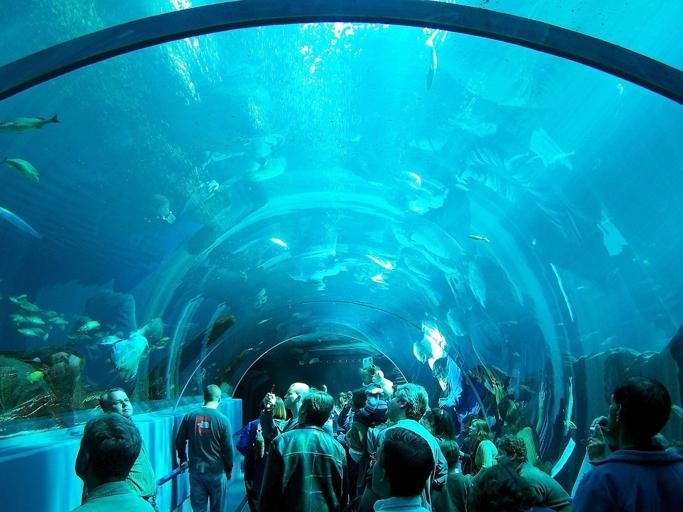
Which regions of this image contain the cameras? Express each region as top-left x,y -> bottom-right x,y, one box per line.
589,423 -> 601,436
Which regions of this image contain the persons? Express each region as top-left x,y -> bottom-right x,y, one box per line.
175,384 -> 234,512
69,385 -> 159,512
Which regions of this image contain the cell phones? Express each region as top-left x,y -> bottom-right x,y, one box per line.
266,383 -> 276,409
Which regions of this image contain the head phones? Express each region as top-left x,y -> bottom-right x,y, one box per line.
369,364 -> 376,383
